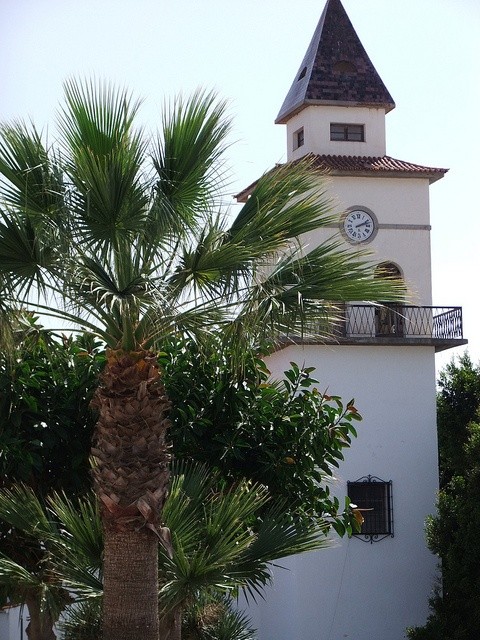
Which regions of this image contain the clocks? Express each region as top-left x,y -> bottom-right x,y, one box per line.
339,205 -> 379,246
